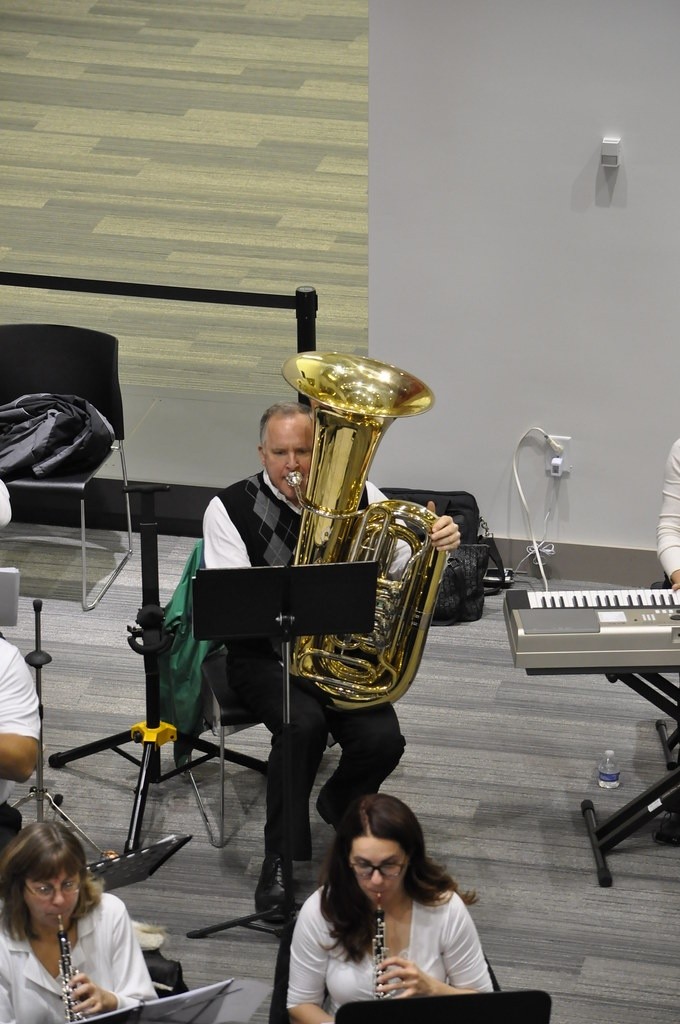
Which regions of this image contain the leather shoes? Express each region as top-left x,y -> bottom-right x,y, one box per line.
254,853 -> 295,924
316,797 -> 337,832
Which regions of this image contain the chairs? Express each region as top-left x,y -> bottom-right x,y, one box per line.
166,536 -> 405,849
0,323 -> 134,612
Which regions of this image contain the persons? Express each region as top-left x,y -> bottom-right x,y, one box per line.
285,793 -> 495,1024
0,639 -> 42,843
656,438 -> 680,591
0,821 -> 158,1024
202,402 -> 460,922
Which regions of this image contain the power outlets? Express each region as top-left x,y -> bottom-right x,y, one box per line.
544,436 -> 575,474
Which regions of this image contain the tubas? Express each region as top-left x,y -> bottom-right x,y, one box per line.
282,349 -> 448,708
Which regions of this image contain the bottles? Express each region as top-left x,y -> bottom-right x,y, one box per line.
598,750 -> 620,788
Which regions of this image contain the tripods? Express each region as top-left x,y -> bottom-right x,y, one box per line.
8,484 -> 380,940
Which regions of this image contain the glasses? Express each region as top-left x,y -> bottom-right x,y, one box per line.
348,857 -> 410,880
24,880 -> 82,901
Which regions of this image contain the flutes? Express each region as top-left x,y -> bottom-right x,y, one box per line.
371,891 -> 393,1000
56,913 -> 88,1022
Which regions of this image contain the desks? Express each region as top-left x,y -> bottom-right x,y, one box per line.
525,663 -> 680,888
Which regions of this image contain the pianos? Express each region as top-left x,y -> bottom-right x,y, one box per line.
504,586 -> 680,888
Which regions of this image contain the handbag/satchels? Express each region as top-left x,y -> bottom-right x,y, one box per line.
379,487 -> 505,596
429,543 -> 491,627
